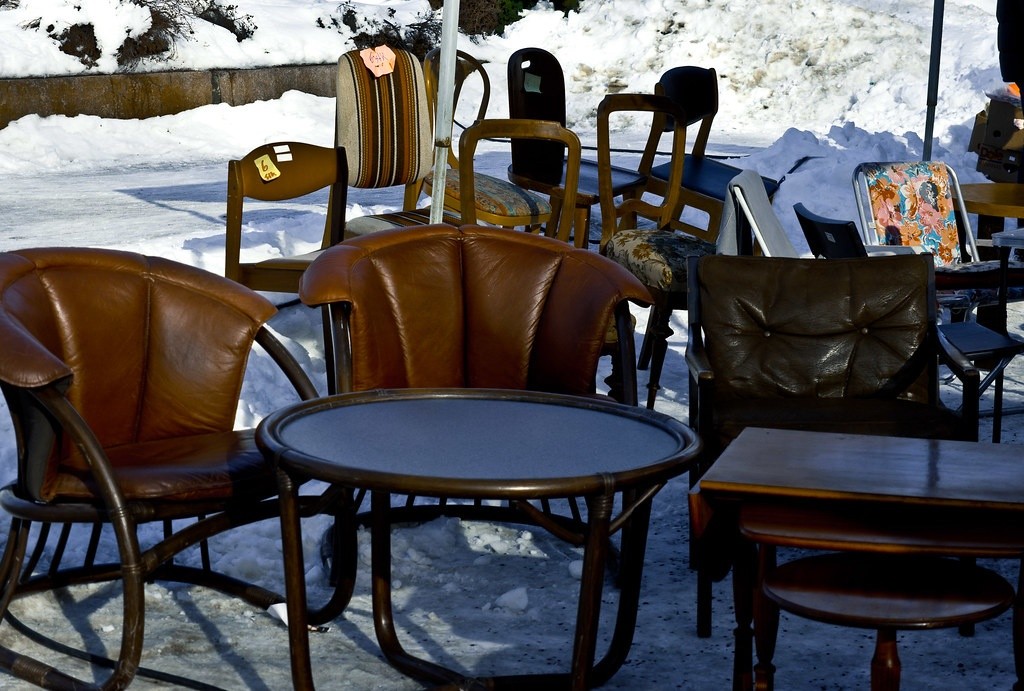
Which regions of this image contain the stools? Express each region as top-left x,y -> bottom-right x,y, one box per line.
763,551 -> 1015,691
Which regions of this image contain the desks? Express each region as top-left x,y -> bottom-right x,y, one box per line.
950,184 -> 1024,239
254,386 -> 703,691
688,427 -> 1024,691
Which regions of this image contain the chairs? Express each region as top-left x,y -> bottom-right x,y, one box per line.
459,120 -> 581,243
297,224 -> 655,691
734,170 -> 800,259
638,66 -> 779,242
416,46 -> 552,235
793,203 -> 869,259
0,247 -> 359,691
852,160 -> 1024,418
597,94 -> 715,410
508,48 -> 648,249
225,142 -> 349,397
684,253 -> 1024,638
322,47 -> 462,247
